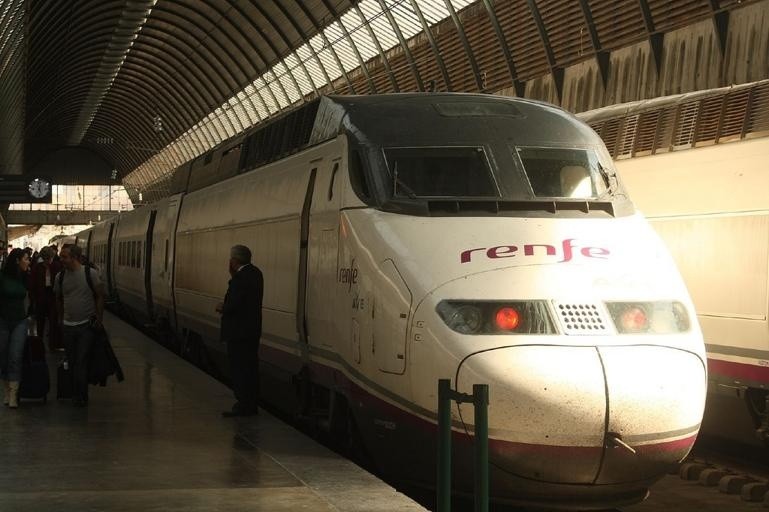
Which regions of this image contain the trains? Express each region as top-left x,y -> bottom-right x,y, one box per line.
46,93 -> 708,495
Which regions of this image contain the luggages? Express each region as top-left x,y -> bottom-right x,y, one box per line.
56,362 -> 74,402
19,336 -> 50,404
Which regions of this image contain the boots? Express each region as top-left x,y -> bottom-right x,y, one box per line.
3,380 -> 10,405
8,381 -> 20,409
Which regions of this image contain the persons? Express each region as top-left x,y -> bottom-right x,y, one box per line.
215,243 -> 266,417
1,243 -> 126,409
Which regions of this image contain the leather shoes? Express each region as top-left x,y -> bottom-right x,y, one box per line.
221,409 -> 258,418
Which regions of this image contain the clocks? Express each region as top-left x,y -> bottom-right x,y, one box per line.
28,178 -> 50,199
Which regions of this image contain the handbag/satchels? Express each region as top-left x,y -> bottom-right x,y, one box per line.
84,317 -> 123,387
